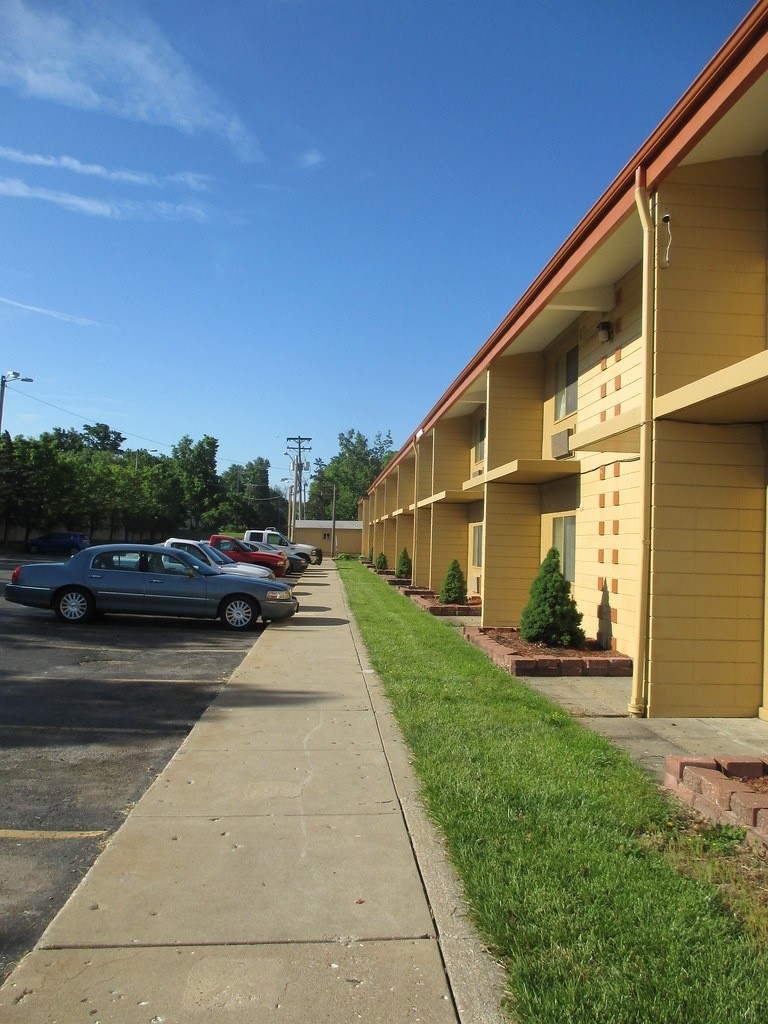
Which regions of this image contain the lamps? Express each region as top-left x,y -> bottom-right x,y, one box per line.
596,321 -> 610,342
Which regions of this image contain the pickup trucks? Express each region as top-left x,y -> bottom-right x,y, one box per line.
112,538 -> 277,581
209,534 -> 287,578
201,530 -> 325,564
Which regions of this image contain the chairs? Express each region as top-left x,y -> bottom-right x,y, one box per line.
149,553 -> 164,572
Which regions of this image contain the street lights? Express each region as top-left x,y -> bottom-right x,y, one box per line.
284,451 -> 298,544
0,372 -> 34,440
281,477 -> 302,521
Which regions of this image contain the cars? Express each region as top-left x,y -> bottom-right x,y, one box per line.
4,544 -> 299,632
27,533 -> 92,556
212,540 -> 308,574
